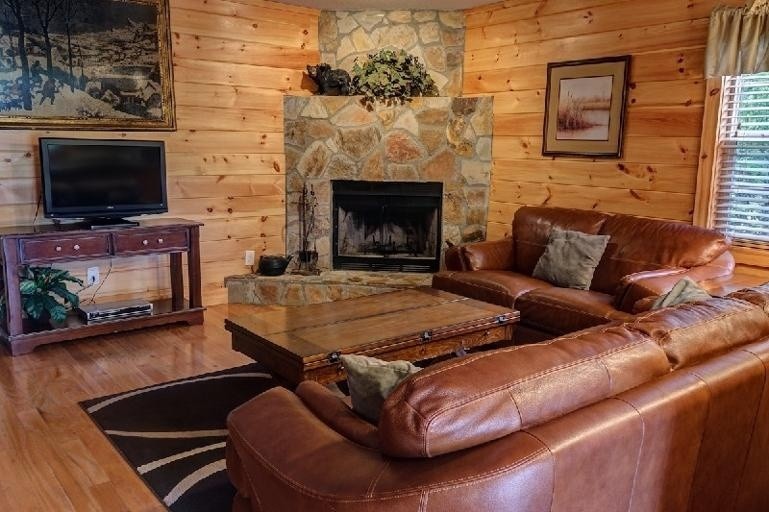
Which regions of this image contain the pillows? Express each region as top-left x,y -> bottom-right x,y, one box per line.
646,272 -> 721,310
332,346 -> 429,422
528,222 -> 614,293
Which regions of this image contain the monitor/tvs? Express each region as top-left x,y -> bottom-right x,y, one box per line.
37,135 -> 167,228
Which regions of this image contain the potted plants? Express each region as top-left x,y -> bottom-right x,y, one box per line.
0,261 -> 86,327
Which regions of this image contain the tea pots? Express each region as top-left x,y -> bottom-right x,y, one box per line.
258,253 -> 294,276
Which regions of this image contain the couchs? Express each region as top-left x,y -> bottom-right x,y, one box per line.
211,272 -> 769,511
430,202 -> 743,345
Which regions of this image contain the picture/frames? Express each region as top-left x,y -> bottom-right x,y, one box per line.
1,1 -> 179,137
540,52 -> 634,160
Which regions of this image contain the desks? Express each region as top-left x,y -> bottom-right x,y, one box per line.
0,212 -> 208,359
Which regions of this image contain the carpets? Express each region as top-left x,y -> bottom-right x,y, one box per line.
76,341 -> 510,512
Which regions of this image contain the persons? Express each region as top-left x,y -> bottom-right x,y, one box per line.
30,60 -> 48,91
39,78 -> 58,105
